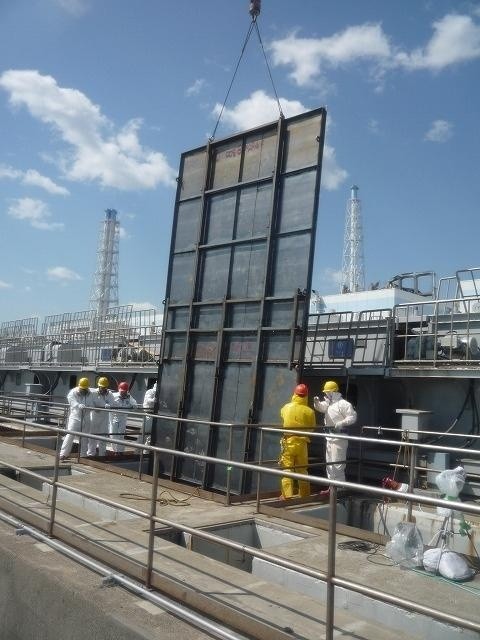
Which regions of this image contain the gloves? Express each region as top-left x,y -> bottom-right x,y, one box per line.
335,421 -> 343,431
104,404 -> 111,408
131,402 -> 139,409
78,403 -> 86,409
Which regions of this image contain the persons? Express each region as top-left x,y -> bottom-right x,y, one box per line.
109,382 -> 140,454
314,380 -> 359,496
86,376 -> 117,459
141,379 -> 158,460
59,376 -> 93,462
280,382 -> 317,500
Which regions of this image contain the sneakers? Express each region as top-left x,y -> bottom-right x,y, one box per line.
320,488 -> 330,496
60,455 -> 68,460
279,495 -> 290,501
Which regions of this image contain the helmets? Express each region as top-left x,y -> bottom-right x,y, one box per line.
78,378 -> 91,390
97,377 -> 109,387
294,384 -> 308,397
322,381 -> 338,392
118,382 -> 128,392
